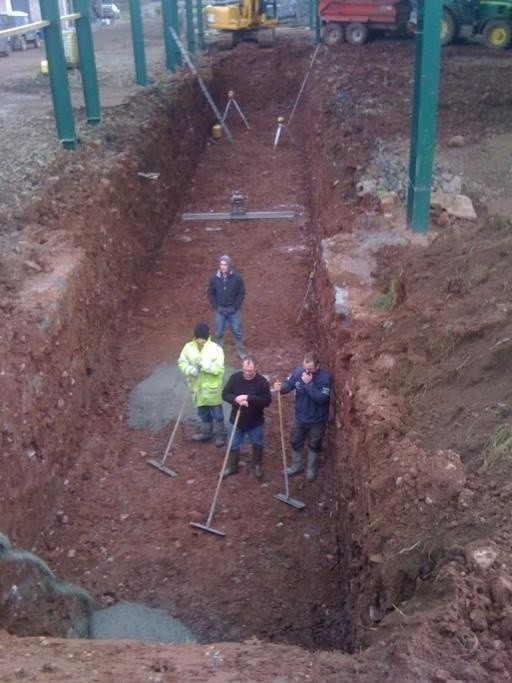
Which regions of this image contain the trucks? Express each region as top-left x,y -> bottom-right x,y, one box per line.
317,0 -> 512,50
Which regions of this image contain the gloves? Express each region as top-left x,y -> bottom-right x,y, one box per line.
190,367 -> 198,376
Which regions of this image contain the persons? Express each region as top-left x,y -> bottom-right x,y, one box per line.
220,359 -> 271,483
271,352 -> 333,483
176,322 -> 230,448
207,255 -> 247,362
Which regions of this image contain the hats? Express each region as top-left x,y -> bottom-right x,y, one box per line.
220,254 -> 232,265
194,322 -> 209,340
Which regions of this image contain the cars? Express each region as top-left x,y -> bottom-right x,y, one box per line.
0,11 -> 41,57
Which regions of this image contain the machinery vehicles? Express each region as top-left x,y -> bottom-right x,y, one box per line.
202,0 -> 279,51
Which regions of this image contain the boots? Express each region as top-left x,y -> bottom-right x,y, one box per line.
191,421 -> 214,442
281,447 -> 304,477
212,419 -> 226,447
218,449 -> 240,478
304,446 -> 320,482
252,446 -> 265,478
